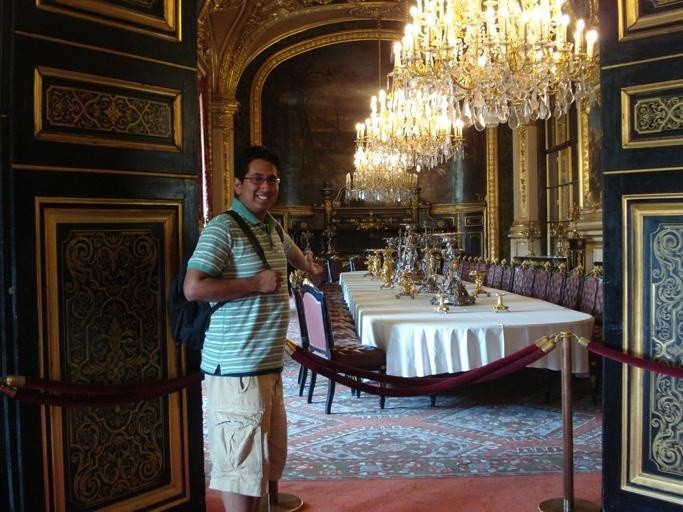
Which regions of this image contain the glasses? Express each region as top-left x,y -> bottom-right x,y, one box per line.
239,176 -> 280,185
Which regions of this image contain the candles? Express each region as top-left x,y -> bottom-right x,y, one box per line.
366,21 -> 467,174
387,1 -> 601,132
346,117 -> 419,204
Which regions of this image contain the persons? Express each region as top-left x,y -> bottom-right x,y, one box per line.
183,147 -> 321,512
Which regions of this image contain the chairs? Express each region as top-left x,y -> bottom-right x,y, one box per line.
520,267 -> 535,296
512,266 -> 524,295
486,261 -> 493,288
546,268 -> 563,301
574,275 -> 598,316
532,268 -> 549,299
493,263 -> 505,290
560,270 -> 580,307
501,264 -> 512,291
298,277 -> 389,415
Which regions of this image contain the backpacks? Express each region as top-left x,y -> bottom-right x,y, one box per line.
167,210 -> 286,349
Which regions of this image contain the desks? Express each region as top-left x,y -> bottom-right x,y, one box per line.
337,266 -> 596,407
